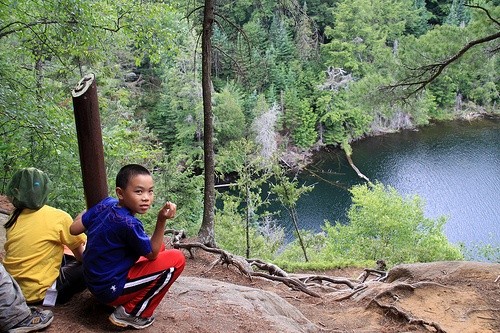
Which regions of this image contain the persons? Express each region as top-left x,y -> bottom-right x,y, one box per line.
0,262 -> 54,333
70,164 -> 186,329
3,167 -> 87,306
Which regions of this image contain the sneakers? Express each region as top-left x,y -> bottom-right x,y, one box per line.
7,306 -> 55,333
108,305 -> 156,330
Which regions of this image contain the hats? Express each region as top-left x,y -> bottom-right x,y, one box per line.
5,167 -> 50,210
